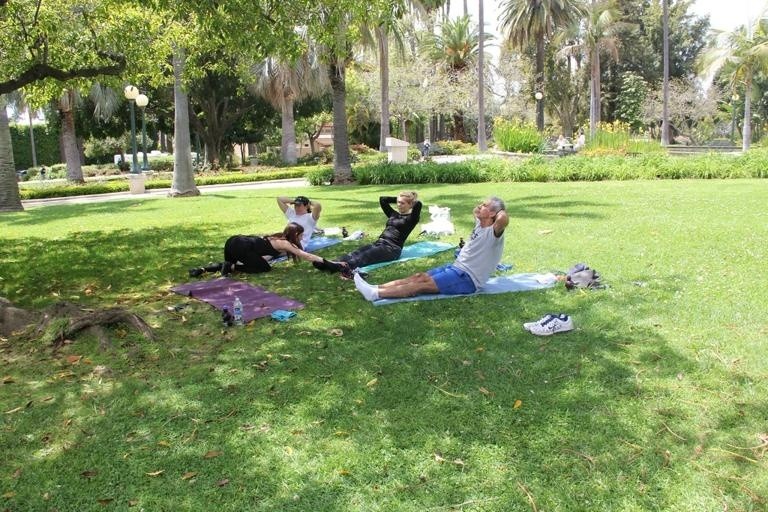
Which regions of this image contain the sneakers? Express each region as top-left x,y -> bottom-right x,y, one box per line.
338,266 -> 370,281
523,313 -> 565,332
529,313 -> 574,337
187,267 -> 203,277
220,260 -> 232,277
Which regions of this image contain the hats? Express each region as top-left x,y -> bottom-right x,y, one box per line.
289,196 -> 310,205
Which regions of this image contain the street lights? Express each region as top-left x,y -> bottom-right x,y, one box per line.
125,85 -> 142,173
136,94 -> 149,170
535,92 -> 544,131
732,94 -> 740,140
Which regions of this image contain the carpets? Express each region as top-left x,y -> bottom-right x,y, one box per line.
270,236 -> 559,306
168,276 -> 304,324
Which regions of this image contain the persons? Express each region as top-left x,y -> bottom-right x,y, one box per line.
354,199 -> 509,303
261,195 -> 322,264
312,191 -> 422,275
555,135 -> 569,150
423,137 -> 432,158
574,132 -> 585,150
190,222 -> 348,278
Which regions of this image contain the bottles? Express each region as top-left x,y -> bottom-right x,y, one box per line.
233,297 -> 243,327
223,306 -> 232,327
455,237 -> 466,257
343,226 -> 348,238
